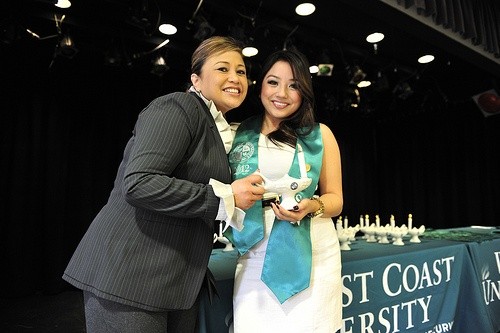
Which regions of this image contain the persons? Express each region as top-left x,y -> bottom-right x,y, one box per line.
228,48 -> 343,333
62,36 -> 265,333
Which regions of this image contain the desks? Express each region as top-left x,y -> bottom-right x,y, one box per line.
199,226 -> 500,333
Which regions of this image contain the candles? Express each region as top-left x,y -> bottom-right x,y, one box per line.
376,215 -> 380,227
390,215 -> 395,227
336,216 -> 342,230
344,216 -> 349,229
408,213 -> 412,229
360,214 -> 364,226
365,215 -> 370,226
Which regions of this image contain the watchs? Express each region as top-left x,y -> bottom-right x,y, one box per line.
306,198 -> 325,219
